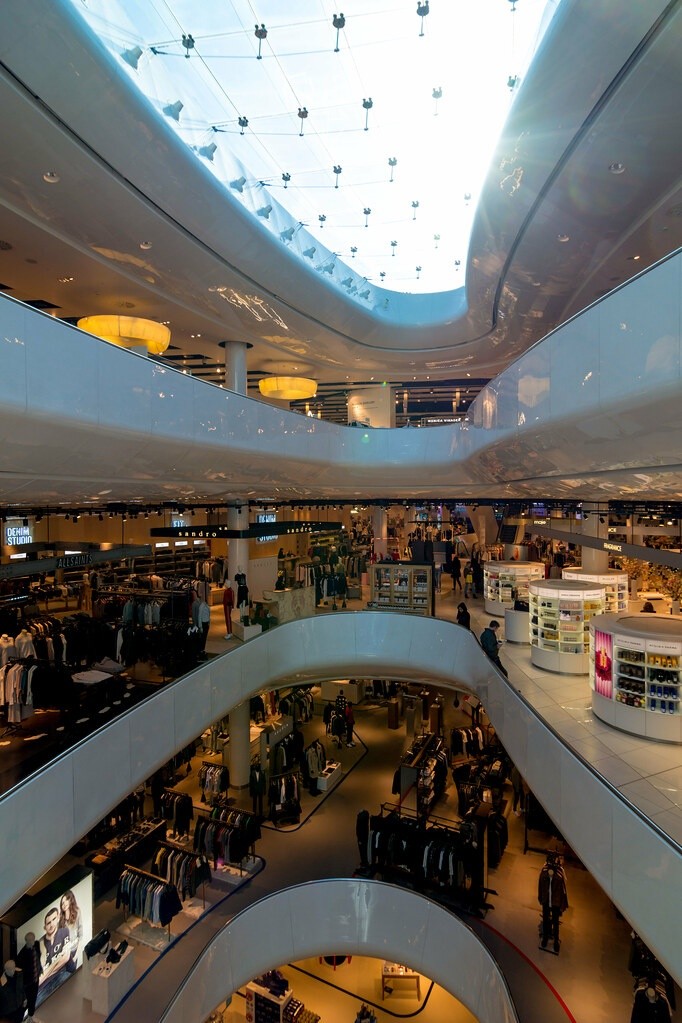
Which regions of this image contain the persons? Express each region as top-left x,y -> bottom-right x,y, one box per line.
278,547 -> 286,559
480,620 -> 507,676
77,574 -> 89,608
372,862 -> 385,881
450,556 -> 484,598
465,792 -> 483,818
23,891 -> 83,1023
336,690 -> 355,748
0,630 -> 37,666
456,602 -> 470,629
538,848 -> 569,953
223,568 -> 249,639
314,538 -> 336,564
354,1006 -> 377,1023
640,602 -> 656,612
348,520 -> 374,546
0,932 -> 43,1023
249,764 -> 267,823
198,602 -> 211,655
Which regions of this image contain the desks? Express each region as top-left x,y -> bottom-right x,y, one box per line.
382,963 -> 421,1002
92,942 -> 137,1017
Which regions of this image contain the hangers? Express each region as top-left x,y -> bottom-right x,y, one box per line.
124,762 -> 254,888
431,821 -> 662,996
297,551 -> 362,579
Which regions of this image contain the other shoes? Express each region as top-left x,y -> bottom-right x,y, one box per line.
351,741 -> 355,745
519,808 -> 530,812
347,743 -> 352,748
465,593 -> 469,597
106,961 -> 112,974
460,586 -> 462,589
473,594 -> 478,598
98,962 -> 105,975
452,588 -> 455,590
512,809 -> 518,816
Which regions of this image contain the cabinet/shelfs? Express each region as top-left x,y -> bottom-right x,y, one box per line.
528,579 -> 607,676
591,614 -> 682,746
296,530 -> 340,555
486,560 -> 546,617
369,563 -> 432,615
562,566 -> 628,614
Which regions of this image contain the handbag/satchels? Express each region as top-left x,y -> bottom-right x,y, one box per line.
85,928 -> 111,960
466,573 -> 472,583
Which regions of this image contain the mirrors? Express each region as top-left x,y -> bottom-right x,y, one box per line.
400,764 -> 419,820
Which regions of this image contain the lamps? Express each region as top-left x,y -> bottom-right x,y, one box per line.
76,292 -> 171,356
258,360 -> 317,399
0,504 -> 682,527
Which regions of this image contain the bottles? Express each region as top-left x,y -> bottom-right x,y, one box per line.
563,646 -> 580,653
620,664 -> 644,679
560,624 -> 577,631
584,646 -> 586,653
600,649 -> 607,667
651,685 -> 677,713
618,649 -> 645,664
648,655 -> 679,669
560,611 -> 571,621
571,613 -> 582,621
541,611 -> 556,618
532,639 -> 538,645
585,612 -> 596,620
564,636 -> 578,642
560,602 -> 579,609
544,644 -> 557,651
619,680 -> 644,694
543,620 -> 557,630
584,601 -> 601,611
541,630 -> 558,640
617,691 -> 645,708
650,669 -> 678,684
